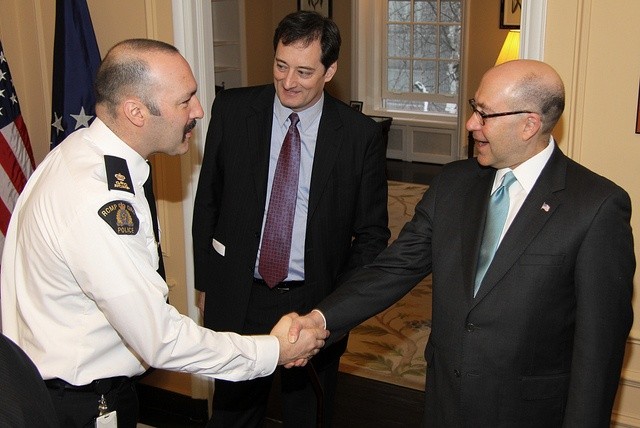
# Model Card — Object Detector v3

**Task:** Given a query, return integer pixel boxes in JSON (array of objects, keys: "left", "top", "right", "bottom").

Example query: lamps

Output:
[{"left": 495, "top": 29, "right": 522, "bottom": 64}]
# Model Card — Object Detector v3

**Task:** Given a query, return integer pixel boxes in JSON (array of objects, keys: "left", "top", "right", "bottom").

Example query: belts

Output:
[
  {"left": 255, "top": 278, "right": 305, "bottom": 294},
  {"left": 44, "top": 378, "right": 122, "bottom": 393}
]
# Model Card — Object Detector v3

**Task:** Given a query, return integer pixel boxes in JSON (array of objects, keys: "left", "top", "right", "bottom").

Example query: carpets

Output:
[{"left": 334, "top": 180, "right": 433, "bottom": 391}]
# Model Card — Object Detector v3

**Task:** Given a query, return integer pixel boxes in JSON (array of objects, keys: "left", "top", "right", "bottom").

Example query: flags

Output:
[
  {"left": 50, "top": 0, "right": 170, "bottom": 304},
  {"left": 0, "top": 41, "right": 37, "bottom": 261}
]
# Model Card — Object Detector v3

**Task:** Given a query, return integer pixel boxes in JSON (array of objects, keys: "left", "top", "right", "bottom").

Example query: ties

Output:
[
  {"left": 142, "top": 159, "right": 170, "bottom": 307},
  {"left": 257, "top": 112, "right": 302, "bottom": 291},
  {"left": 473, "top": 170, "right": 517, "bottom": 299}
]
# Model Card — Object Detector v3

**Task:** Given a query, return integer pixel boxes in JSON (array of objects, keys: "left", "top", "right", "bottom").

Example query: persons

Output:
[
  {"left": 1, "top": 40, "right": 332, "bottom": 428},
  {"left": 285, "top": 60, "right": 636, "bottom": 428},
  {"left": 191, "top": 10, "right": 391, "bottom": 428}
]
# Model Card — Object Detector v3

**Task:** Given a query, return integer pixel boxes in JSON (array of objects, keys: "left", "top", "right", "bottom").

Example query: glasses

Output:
[{"left": 469, "top": 98, "right": 542, "bottom": 125}]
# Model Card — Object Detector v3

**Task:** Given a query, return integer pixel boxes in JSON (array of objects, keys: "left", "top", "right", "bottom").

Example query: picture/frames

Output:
[
  {"left": 499, "top": 0, "right": 521, "bottom": 30},
  {"left": 297, "top": 0, "right": 334, "bottom": 21}
]
